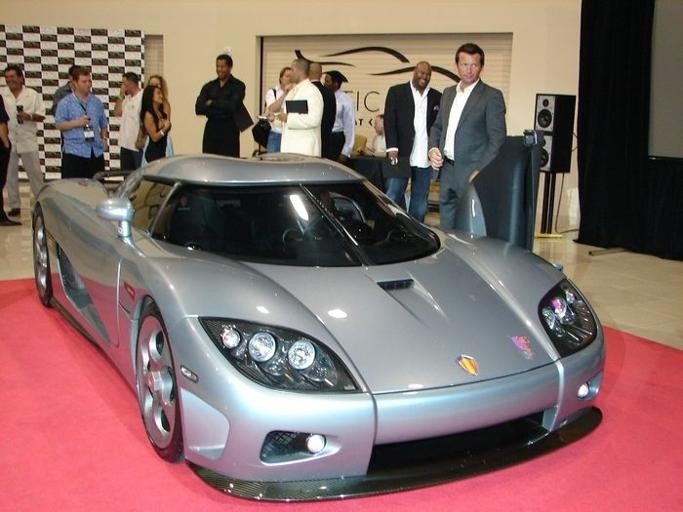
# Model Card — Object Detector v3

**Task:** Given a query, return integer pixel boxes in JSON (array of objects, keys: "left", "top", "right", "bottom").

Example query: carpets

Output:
[{"left": 1, "top": 275, "right": 683, "bottom": 507}]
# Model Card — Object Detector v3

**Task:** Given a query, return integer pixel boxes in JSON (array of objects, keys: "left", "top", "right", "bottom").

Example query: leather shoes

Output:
[
  {"left": 0, "top": 219, "right": 22, "bottom": 226},
  {"left": 8, "top": 208, "right": 20, "bottom": 217}
]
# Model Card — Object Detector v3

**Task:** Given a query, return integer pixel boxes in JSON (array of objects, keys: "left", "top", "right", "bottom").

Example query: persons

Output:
[
  {"left": 428, "top": 43, "right": 507, "bottom": 230},
  {"left": 268, "top": 59, "right": 324, "bottom": 158},
  {"left": 309, "top": 63, "right": 336, "bottom": 157},
  {"left": 114, "top": 72, "right": 144, "bottom": 170},
  {"left": 266, "top": 67, "right": 294, "bottom": 153},
  {"left": 0, "top": 94, "right": 22, "bottom": 227},
  {"left": 195, "top": 54, "right": 246, "bottom": 158},
  {"left": 324, "top": 70, "right": 355, "bottom": 163},
  {"left": 383, "top": 61, "right": 442, "bottom": 224},
  {"left": 141, "top": 75, "right": 174, "bottom": 167},
  {"left": 140, "top": 85, "right": 172, "bottom": 163},
  {"left": 0, "top": 66, "right": 45, "bottom": 216},
  {"left": 358, "top": 114, "right": 387, "bottom": 157},
  {"left": 53, "top": 65, "right": 82, "bottom": 114},
  {"left": 55, "top": 70, "right": 109, "bottom": 178}
]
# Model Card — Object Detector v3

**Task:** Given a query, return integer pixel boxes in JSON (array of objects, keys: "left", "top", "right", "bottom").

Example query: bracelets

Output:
[
  {"left": 160, "top": 130, "right": 164, "bottom": 136},
  {"left": 118, "top": 95, "right": 123, "bottom": 100}
]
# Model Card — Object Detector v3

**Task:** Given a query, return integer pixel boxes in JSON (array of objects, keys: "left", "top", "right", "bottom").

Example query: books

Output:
[
  {"left": 285, "top": 99, "right": 309, "bottom": 114},
  {"left": 233, "top": 103, "right": 253, "bottom": 132}
]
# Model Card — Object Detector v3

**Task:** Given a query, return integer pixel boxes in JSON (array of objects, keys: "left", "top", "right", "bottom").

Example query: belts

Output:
[{"left": 444, "top": 155, "right": 455, "bottom": 166}]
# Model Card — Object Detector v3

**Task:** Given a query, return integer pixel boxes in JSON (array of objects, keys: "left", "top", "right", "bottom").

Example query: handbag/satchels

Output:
[{"left": 252, "top": 88, "right": 276, "bottom": 149}]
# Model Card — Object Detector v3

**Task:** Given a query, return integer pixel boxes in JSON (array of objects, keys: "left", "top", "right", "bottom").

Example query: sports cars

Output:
[{"left": 29, "top": 150, "right": 607, "bottom": 504}]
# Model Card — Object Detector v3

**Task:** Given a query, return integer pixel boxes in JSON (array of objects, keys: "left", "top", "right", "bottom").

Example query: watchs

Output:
[{"left": 28, "top": 112, "right": 34, "bottom": 121}]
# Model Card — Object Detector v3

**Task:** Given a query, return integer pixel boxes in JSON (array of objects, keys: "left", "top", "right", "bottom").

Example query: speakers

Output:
[{"left": 533, "top": 93, "right": 576, "bottom": 174}]
